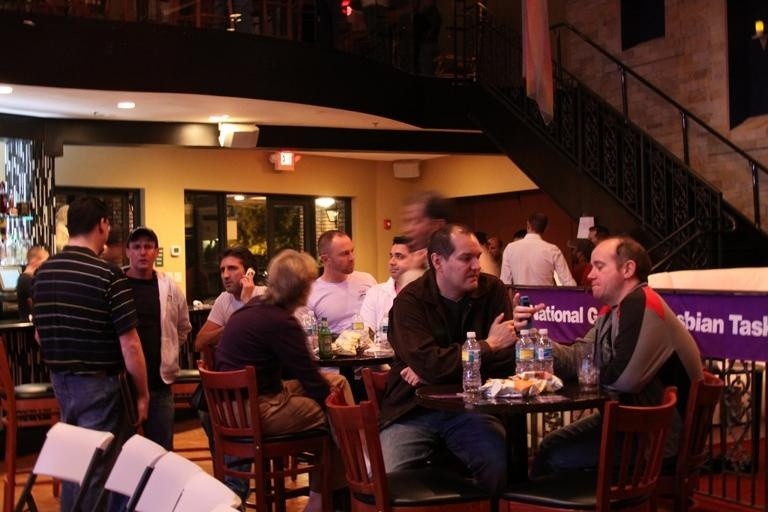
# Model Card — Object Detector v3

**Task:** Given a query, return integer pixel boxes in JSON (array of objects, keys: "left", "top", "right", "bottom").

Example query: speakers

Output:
[{"left": 218, "top": 124, "right": 260, "bottom": 148}]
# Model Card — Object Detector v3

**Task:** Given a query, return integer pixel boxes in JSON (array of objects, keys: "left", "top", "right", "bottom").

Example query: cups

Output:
[{"left": 573, "top": 343, "right": 599, "bottom": 392}]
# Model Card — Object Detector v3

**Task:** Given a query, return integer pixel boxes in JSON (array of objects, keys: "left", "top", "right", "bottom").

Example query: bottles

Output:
[
  {"left": 0, "top": 181, "right": 9, "bottom": 214},
  {"left": 532, "top": 329, "right": 555, "bottom": 373},
  {"left": 461, "top": 331, "right": 480, "bottom": 391},
  {"left": 303, "top": 309, "right": 388, "bottom": 360},
  {"left": 515, "top": 328, "right": 532, "bottom": 372}
]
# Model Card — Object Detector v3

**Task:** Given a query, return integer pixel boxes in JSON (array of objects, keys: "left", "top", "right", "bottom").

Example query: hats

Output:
[{"left": 127, "top": 227, "right": 158, "bottom": 247}]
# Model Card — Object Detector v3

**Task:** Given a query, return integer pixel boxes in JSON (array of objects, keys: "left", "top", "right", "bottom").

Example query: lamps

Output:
[{"left": 270, "top": 151, "right": 296, "bottom": 172}]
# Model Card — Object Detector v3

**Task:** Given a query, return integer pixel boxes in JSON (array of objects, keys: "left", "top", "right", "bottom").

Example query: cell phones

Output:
[
  {"left": 245, "top": 268, "right": 256, "bottom": 278},
  {"left": 520, "top": 296, "right": 532, "bottom": 330}
]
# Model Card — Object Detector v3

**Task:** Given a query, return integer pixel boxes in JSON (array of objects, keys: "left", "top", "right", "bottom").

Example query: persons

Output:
[
  {"left": 192, "top": 246, "right": 270, "bottom": 500},
  {"left": 29, "top": 195, "right": 153, "bottom": 511},
  {"left": 299, "top": 189, "right": 623, "bottom": 388},
  {"left": 207, "top": 247, "right": 359, "bottom": 512},
  {"left": 15, "top": 245, "right": 51, "bottom": 322},
  {"left": 118, "top": 224, "right": 193, "bottom": 455},
  {"left": 511, "top": 232, "right": 708, "bottom": 499},
  {"left": 359, "top": 224, "right": 532, "bottom": 503}
]
